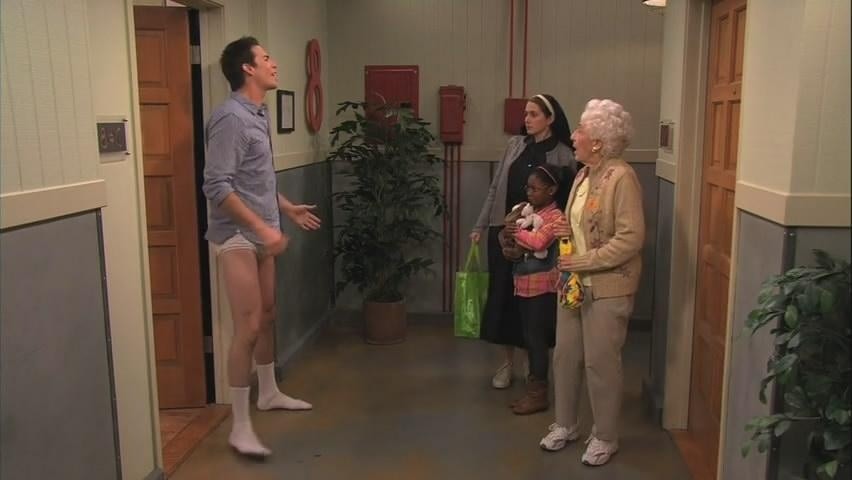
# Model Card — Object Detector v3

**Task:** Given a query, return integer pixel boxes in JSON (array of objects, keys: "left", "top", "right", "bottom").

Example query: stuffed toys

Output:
[
  {"left": 497, "top": 201, "right": 531, "bottom": 262},
  {"left": 514, "top": 203, "right": 549, "bottom": 260}
]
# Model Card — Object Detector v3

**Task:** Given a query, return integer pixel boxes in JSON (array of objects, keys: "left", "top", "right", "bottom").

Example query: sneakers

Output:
[
  {"left": 582, "top": 433, "right": 618, "bottom": 465},
  {"left": 540, "top": 416, "right": 580, "bottom": 451},
  {"left": 493, "top": 361, "right": 513, "bottom": 389}
]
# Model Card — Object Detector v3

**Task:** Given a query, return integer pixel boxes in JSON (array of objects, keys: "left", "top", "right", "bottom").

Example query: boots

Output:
[{"left": 508, "top": 373, "right": 548, "bottom": 414}]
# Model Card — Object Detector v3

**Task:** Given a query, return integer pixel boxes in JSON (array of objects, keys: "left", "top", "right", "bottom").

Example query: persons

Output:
[
  {"left": 537, "top": 98, "right": 647, "bottom": 468},
  {"left": 201, "top": 36, "right": 322, "bottom": 458},
  {"left": 498, "top": 161, "right": 567, "bottom": 416},
  {"left": 472, "top": 91, "right": 581, "bottom": 388}
]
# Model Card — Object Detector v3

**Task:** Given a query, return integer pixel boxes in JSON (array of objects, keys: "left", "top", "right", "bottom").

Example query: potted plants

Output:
[{"left": 325, "top": 89, "right": 451, "bottom": 347}]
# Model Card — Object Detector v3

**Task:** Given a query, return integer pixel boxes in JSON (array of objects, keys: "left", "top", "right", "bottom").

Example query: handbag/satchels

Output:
[
  {"left": 455, "top": 270, "right": 490, "bottom": 338},
  {"left": 559, "top": 274, "right": 585, "bottom": 310}
]
